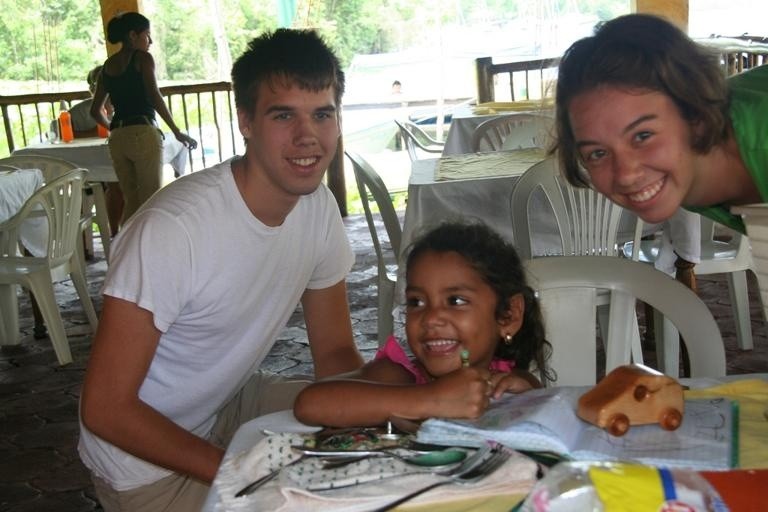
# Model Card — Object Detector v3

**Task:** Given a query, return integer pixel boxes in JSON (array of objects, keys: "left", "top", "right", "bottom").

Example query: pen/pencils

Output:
[{"left": 461, "top": 350, "right": 470, "bottom": 367}]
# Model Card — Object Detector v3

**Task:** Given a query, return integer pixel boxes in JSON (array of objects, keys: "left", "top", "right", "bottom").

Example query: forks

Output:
[
  {"left": 371, "top": 443, "right": 514, "bottom": 512},
  {"left": 306, "top": 443, "right": 492, "bottom": 498}
]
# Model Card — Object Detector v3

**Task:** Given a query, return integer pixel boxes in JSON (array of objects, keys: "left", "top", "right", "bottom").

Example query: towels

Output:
[{"left": 234, "top": 422, "right": 537, "bottom": 512}]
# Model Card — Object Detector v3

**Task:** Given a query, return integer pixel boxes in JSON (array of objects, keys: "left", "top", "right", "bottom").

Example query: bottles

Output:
[
  {"left": 59, "top": 99, "right": 73, "bottom": 142},
  {"left": 97, "top": 103, "right": 108, "bottom": 137}
]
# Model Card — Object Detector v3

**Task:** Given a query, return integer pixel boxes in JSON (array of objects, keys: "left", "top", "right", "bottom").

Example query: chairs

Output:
[
  {"left": 627, "top": 218, "right": 760, "bottom": 373},
  {"left": 522, "top": 255, "right": 726, "bottom": 381},
  {"left": 510, "top": 158, "right": 643, "bottom": 375},
  {"left": 344, "top": 150, "right": 404, "bottom": 350},
  {"left": 394, "top": 117, "right": 445, "bottom": 160},
  {"left": 715, "top": 44, "right": 768, "bottom": 78},
  {"left": 1, "top": 166, "right": 98, "bottom": 366},
  {"left": 478, "top": 56, "right": 561, "bottom": 100},
  {"left": 472, "top": 113, "right": 558, "bottom": 153},
  {"left": 1, "top": 156, "right": 112, "bottom": 267}
]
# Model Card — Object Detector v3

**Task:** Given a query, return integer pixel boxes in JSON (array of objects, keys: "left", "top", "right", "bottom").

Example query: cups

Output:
[{"left": 48, "top": 119, "right": 59, "bottom": 143}]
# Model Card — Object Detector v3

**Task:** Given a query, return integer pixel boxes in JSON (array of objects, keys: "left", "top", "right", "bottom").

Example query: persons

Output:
[
  {"left": 76, "top": 28, "right": 364, "bottom": 512},
  {"left": 294, "top": 218, "right": 558, "bottom": 428},
  {"left": 90, "top": 11, "right": 197, "bottom": 232},
  {"left": 54, "top": 66, "right": 125, "bottom": 264},
  {"left": 554, "top": 14, "right": 768, "bottom": 241},
  {"left": 391, "top": 80, "right": 403, "bottom": 97}
]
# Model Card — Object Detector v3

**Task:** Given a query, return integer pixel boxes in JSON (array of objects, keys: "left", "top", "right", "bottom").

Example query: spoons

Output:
[{"left": 290, "top": 444, "right": 467, "bottom": 467}]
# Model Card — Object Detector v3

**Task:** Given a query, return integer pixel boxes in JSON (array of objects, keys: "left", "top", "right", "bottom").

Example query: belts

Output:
[{"left": 109, "top": 117, "right": 156, "bottom": 129}]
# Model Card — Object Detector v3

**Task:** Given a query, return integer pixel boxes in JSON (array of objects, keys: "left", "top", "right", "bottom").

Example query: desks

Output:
[
  {"left": 441, "top": 98, "right": 561, "bottom": 157},
  {"left": 11, "top": 131, "right": 190, "bottom": 261},
  {"left": 1, "top": 165, "right": 45, "bottom": 259},
  {"left": 401, "top": 148, "right": 702, "bottom": 294},
  {"left": 201, "top": 374, "right": 768, "bottom": 512}
]
